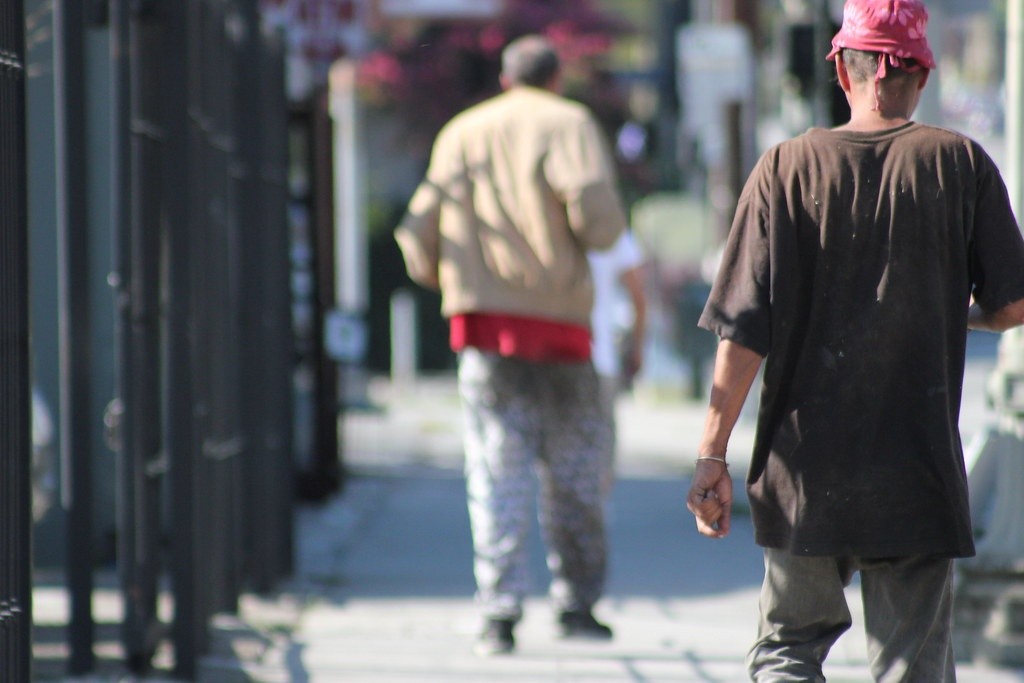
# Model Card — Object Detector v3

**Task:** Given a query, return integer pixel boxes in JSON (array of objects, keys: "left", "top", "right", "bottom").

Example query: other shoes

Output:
[
  {"left": 472, "top": 620, "right": 514, "bottom": 656},
  {"left": 554, "top": 611, "right": 613, "bottom": 641}
]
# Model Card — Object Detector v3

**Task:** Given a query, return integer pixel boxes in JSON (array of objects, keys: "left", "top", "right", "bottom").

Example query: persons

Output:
[
  {"left": 683, "top": 0, "right": 1024, "bottom": 683},
  {"left": 394, "top": 35, "right": 645, "bottom": 657}
]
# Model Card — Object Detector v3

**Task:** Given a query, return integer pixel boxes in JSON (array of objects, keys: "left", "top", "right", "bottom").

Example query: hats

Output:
[{"left": 827, "top": 0, "right": 935, "bottom": 112}]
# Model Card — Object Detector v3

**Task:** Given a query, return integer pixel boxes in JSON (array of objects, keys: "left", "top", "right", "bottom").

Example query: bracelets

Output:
[{"left": 695, "top": 457, "right": 726, "bottom": 464}]
